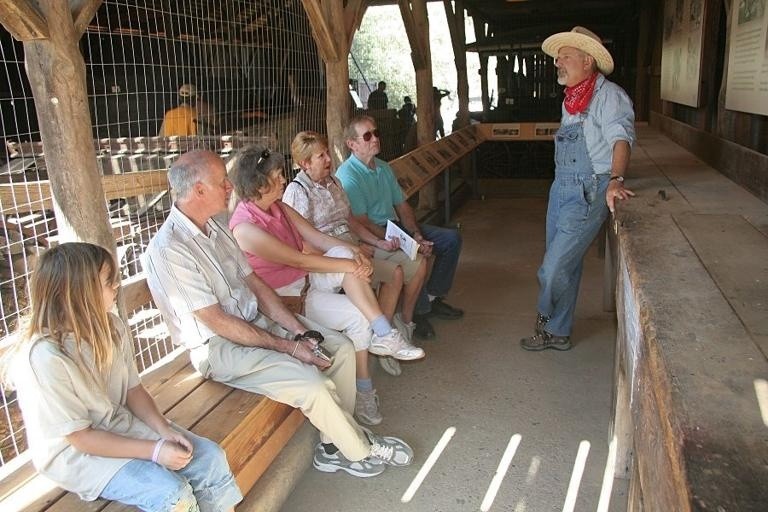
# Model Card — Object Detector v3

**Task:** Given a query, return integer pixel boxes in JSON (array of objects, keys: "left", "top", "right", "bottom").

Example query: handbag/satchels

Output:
[{"left": 278, "top": 295, "right": 305, "bottom": 319}]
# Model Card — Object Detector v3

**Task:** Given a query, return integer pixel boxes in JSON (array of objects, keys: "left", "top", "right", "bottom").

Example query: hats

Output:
[
  {"left": 179, "top": 84, "right": 199, "bottom": 99},
  {"left": 542, "top": 27, "right": 614, "bottom": 76}
]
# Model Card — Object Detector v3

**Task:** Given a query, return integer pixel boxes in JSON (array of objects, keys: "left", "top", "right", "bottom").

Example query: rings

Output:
[{"left": 364, "top": 266, "right": 369, "bottom": 270}]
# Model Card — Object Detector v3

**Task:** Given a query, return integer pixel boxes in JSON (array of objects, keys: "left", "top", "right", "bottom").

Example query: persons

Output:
[
  {"left": 3, "top": 242, "right": 245, "bottom": 511},
  {"left": 403, "top": 96, "right": 415, "bottom": 115},
  {"left": 368, "top": 81, "right": 389, "bottom": 110},
  {"left": 452, "top": 111, "right": 461, "bottom": 132},
  {"left": 518, "top": 25, "right": 636, "bottom": 351},
  {"left": 158, "top": 83, "right": 210, "bottom": 137},
  {"left": 433, "top": 87, "right": 450, "bottom": 139},
  {"left": 281, "top": 131, "right": 432, "bottom": 377},
  {"left": 333, "top": 113, "right": 464, "bottom": 339},
  {"left": 228, "top": 143, "right": 426, "bottom": 428},
  {"left": 141, "top": 149, "right": 415, "bottom": 478}
]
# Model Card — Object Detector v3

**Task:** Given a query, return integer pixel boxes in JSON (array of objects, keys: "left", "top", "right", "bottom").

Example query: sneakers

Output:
[
  {"left": 368, "top": 330, "right": 426, "bottom": 361},
  {"left": 429, "top": 298, "right": 464, "bottom": 319},
  {"left": 536, "top": 311, "right": 550, "bottom": 334},
  {"left": 414, "top": 316, "right": 435, "bottom": 342},
  {"left": 354, "top": 387, "right": 383, "bottom": 424},
  {"left": 391, "top": 313, "right": 416, "bottom": 342},
  {"left": 358, "top": 425, "right": 415, "bottom": 468},
  {"left": 520, "top": 331, "right": 572, "bottom": 352},
  {"left": 312, "top": 442, "right": 385, "bottom": 478},
  {"left": 378, "top": 356, "right": 402, "bottom": 377}
]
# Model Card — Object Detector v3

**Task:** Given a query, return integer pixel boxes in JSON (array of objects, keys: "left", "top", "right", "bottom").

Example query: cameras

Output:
[{"left": 312, "top": 345, "right": 332, "bottom": 361}]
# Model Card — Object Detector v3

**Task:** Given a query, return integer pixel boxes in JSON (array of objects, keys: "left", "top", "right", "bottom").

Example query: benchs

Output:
[{"left": 0, "top": 273, "right": 306, "bottom": 512}]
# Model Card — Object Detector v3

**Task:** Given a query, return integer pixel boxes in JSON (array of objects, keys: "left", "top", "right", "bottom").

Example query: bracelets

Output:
[
  {"left": 291, "top": 340, "right": 300, "bottom": 358},
  {"left": 152, "top": 439, "right": 165, "bottom": 463},
  {"left": 410, "top": 229, "right": 420, "bottom": 237}
]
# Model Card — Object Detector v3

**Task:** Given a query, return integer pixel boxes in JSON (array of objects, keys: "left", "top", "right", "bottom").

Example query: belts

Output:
[{"left": 325, "top": 223, "right": 351, "bottom": 237}]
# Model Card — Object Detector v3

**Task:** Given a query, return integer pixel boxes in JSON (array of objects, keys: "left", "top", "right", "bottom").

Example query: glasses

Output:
[
  {"left": 354, "top": 128, "right": 379, "bottom": 142},
  {"left": 256, "top": 148, "right": 272, "bottom": 165},
  {"left": 294, "top": 330, "right": 324, "bottom": 344}
]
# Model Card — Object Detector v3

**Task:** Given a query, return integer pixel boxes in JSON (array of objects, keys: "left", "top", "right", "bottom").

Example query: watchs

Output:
[{"left": 609, "top": 175, "right": 624, "bottom": 183}]
